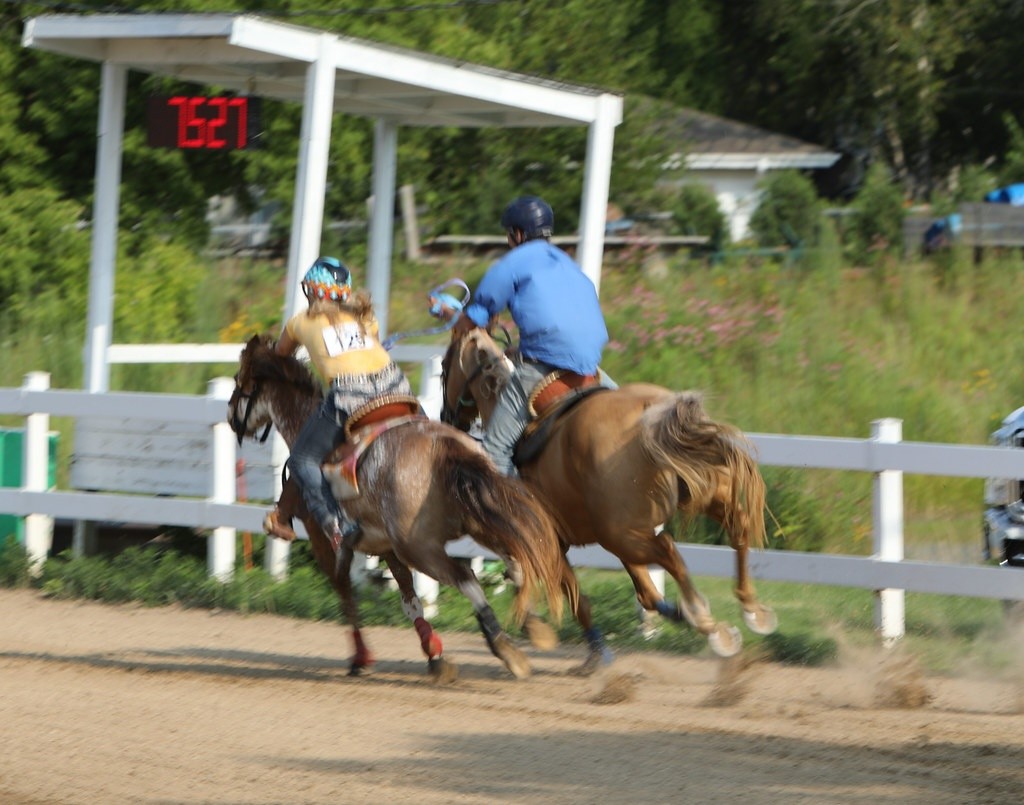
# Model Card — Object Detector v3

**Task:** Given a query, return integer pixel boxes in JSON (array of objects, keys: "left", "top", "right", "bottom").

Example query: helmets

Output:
[
  {"left": 304, "top": 256, "right": 352, "bottom": 304},
  {"left": 501, "top": 195, "right": 554, "bottom": 240}
]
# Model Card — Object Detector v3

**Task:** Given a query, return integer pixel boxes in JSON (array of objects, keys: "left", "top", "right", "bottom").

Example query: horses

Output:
[
  {"left": 438, "top": 301, "right": 782, "bottom": 658},
  {"left": 227, "top": 313, "right": 609, "bottom": 691}
]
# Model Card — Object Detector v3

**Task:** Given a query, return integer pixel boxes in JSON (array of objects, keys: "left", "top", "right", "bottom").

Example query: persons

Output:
[
  {"left": 275, "top": 257, "right": 413, "bottom": 572},
  {"left": 429, "top": 195, "right": 609, "bottom": 476}
]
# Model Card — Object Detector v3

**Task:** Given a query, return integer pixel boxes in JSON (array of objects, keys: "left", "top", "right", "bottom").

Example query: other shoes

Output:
[{"left": 321, "top": 508, "right": 365, "bottom": 581}]
